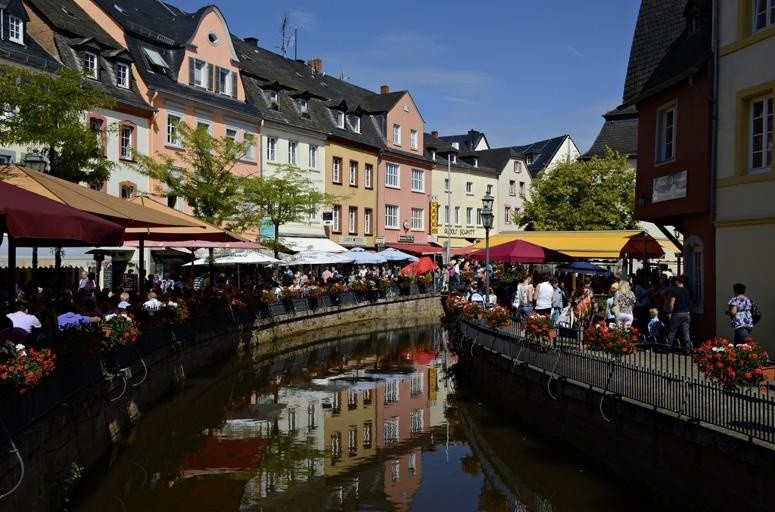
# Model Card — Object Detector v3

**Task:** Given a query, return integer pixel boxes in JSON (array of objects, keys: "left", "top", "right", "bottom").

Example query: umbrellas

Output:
[
  {"left": 0, "top": 157, "right": 211, "bottom": 235},
  {"left": 134, "top": 213, "right": 421, "bottom": 270},
  {"left": 115, "top": 192, "right": 252, "bottom": 296},
  {"left": 462, "top": 239, "right": 581, "bottom": 264},
  {"left": 0, "top": 179, "right": 127, "bottom": 249}
]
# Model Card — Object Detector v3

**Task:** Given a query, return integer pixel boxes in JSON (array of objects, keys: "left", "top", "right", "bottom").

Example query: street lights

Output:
[{"left": 480, "top": 190, "right": 494, "bottom": 307}]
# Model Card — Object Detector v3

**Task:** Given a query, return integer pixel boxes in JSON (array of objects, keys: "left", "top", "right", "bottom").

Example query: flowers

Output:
[
  {"left": 1, "top": 277, "right": 437, "bottom": 387},
  {"left": 688, "top": 336, "right": 768, "bottom": 388},
  {"left": 440, "top": 277, "right": 643, "bottom": 359}
]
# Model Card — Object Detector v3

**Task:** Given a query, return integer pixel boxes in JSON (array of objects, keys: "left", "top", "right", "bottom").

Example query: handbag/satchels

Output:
[
  {"left": 511, "top": 288, "right": 521, "bottom": 309},
  {"left": 751, "top": 305, "right": 764, "bottom": 326}
]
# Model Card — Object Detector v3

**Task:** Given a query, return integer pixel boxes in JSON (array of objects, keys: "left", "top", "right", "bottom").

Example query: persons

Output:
[
  {"left": 1, "top": 258, "right": 698, "bottom": 380},
  {"left": 725, "top": 281, "right": 757, "bottom": 347},
  {"left": 515, "top": 266, "right": 697, "bottom": 355},
  {"left": 405, "top": 259, "right": 500, "bottom": 311},
  {"left": 238, "top": 262, "right": 403, "bottom": 295},
  {"left": 1, "top": 267, "right": 237, "bottom": 346}
]
